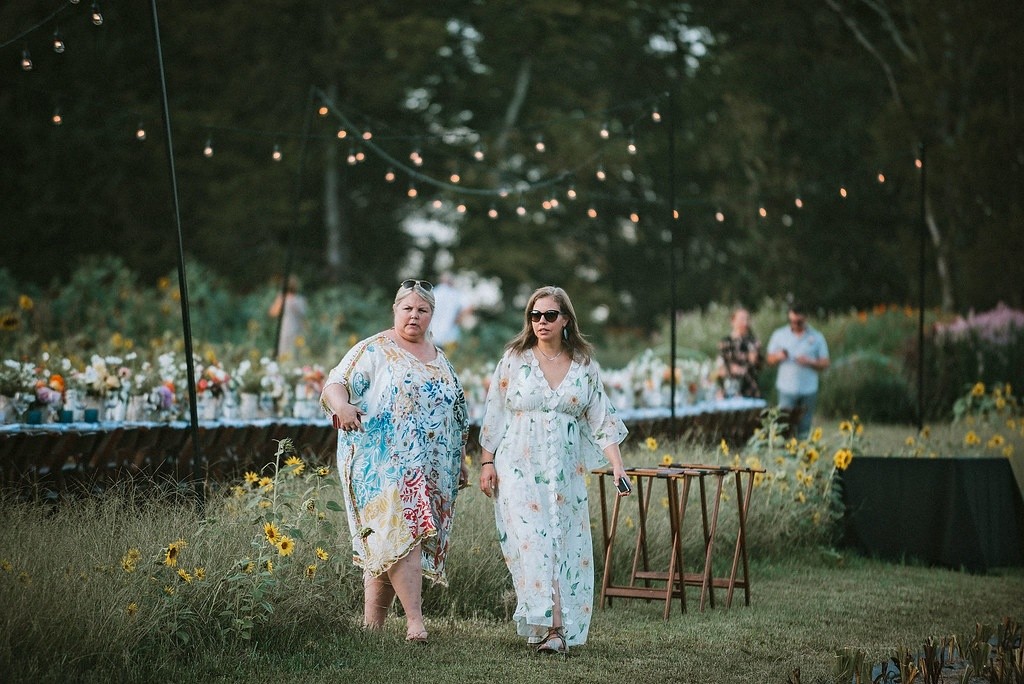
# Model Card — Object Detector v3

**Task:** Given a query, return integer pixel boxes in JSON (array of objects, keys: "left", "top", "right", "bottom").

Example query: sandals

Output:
[{"left": 537, "top": 626, "right": 569, "bottom": 654}]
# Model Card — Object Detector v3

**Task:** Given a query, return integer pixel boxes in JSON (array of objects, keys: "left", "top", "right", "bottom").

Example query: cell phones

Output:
[{"left": 613, "top": 477, "right": 631, "bottom": 494}]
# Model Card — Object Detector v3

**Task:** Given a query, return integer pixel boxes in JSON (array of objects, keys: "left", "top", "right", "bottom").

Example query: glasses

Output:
[
  {"left": 528, "top": 310, "right": 566, "bottom": 323},
  {"left": 401, "top": 279, "right": 434, "bottom": 293}
]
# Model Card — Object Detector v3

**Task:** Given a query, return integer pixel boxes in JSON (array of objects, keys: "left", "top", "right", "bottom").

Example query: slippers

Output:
[{"left": 406, "top": 630, "right": 427, "bottom": 641}]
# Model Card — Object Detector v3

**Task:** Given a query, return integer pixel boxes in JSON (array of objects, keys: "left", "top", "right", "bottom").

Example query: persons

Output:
[
  {"left": 428, "top": 268, "right": 472, "bottom": 361},
  {"left": 478, "top": 284, "right": 634, "bottom": 653},
  {"left": 764, "top": 302, "right": 832, "bottom": 443},
  {"left": 318, "top": 278, "right": 471, "bottom": 644},
  {"left": 269, "top": 272, "right": 309, "bottom": 362},
  {"left": 717, "top": 307, "right": 764, "bottom": 446}
]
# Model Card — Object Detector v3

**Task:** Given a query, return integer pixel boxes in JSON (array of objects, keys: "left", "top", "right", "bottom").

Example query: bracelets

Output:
[{"left": 482, "top": 461, "right": 495, "bottom": 467}]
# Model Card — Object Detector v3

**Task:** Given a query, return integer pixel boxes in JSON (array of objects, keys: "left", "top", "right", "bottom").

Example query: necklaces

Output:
[{"left": 535, "top": 344, "right": 566, "bottom": 361}]
